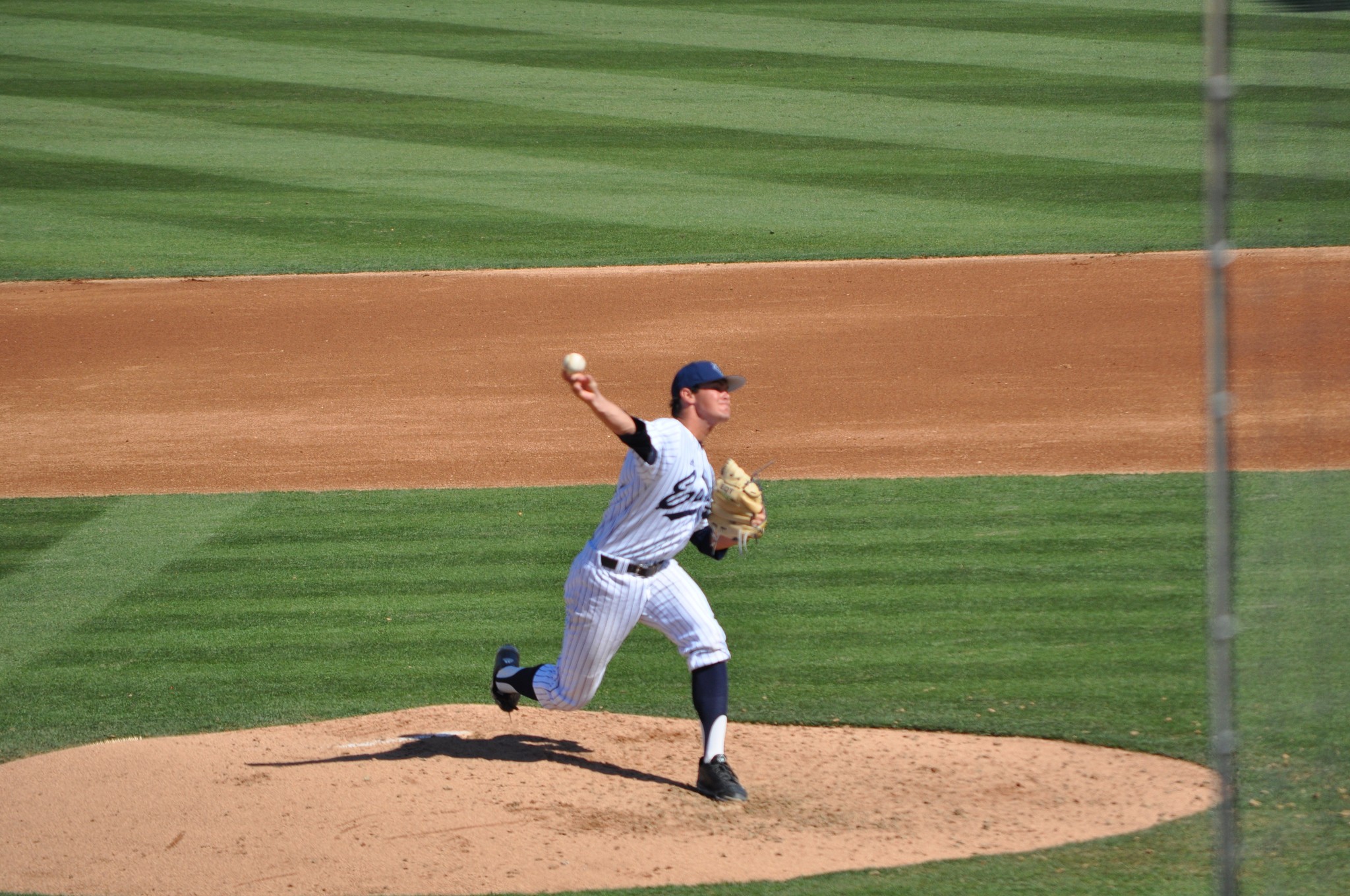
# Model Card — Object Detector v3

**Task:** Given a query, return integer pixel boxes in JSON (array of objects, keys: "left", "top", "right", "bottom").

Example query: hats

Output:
[{"left": 671, "top": 361, "right": 747, "bottom": 397}]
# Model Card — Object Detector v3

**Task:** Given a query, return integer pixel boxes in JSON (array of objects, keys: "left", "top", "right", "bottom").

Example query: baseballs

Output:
[{"left": 563, "top": 353, "right": 586, "bottom": 372}]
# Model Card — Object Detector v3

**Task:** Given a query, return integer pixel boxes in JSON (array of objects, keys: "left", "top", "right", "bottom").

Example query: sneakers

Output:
[
  {"left": 490, "top": 645, "right": 520, "bottom": 713},
  {"left": 696, "top": 754, "right": 747, "bottom": 802}
]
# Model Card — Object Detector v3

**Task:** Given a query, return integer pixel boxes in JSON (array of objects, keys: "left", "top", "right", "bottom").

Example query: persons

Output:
[{"left": 492, "top": 359, "right": 767, "bottom": 804}]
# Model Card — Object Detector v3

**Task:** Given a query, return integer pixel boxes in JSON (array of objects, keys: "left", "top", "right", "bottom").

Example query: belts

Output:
[{"left": 600, "top": 553, "right": 666, "bottom": 577}]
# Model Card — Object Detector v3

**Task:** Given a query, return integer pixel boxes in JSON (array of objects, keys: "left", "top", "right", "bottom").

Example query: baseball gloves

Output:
[{"left": 708, "top": 457, "right": 766, "bottom": 542}]
